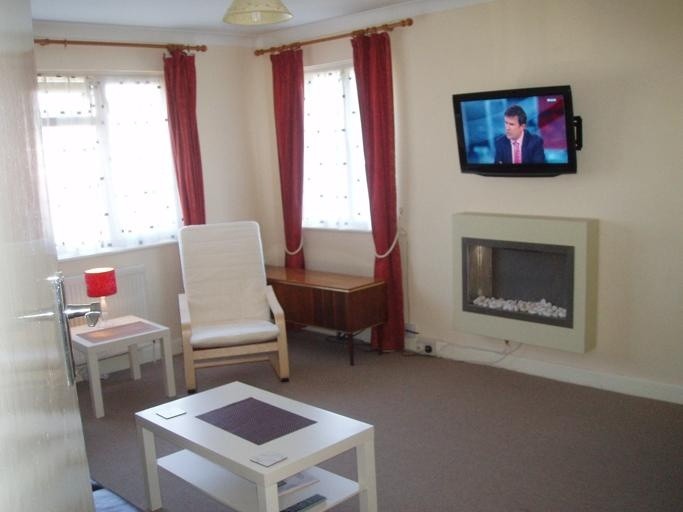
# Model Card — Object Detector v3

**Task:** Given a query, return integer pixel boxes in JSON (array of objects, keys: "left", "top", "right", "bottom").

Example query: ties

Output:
[{"left": 512, "top": 141, "right": 521, "bottom": 164}]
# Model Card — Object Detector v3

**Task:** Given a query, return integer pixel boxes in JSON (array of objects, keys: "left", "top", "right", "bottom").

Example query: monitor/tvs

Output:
[{"left": 452, "top": 85, "right": 582, "bottom": 178}]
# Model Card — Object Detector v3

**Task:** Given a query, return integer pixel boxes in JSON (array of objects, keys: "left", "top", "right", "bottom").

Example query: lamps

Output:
[
  {"left": 222, "top": 1, "right": 293, "bottom": 26},
  {"left": 86, "top": 268, "right": 120, "bottom": 322}
]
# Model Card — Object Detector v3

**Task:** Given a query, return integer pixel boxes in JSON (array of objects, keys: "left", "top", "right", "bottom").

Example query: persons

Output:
[{"left": 495, "top": 105, "right": 545, "bottom": 164}]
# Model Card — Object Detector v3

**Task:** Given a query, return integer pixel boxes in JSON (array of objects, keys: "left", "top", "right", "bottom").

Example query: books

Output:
[{"left": 278, "top": 470, "right": 320, "bottom": 496}]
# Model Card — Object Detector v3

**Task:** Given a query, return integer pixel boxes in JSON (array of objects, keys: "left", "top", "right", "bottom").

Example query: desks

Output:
[
  {"left": 266, "top": 265, "right": 388, "bottom": 365},
  {"left": 72, "top": 314, "right": 178, "bottom": 419},
  {"left": 136, "top": 379, "right": 377, "bottom": 511}
]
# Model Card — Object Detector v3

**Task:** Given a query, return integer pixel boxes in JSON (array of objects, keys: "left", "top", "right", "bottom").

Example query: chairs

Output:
[{"left": 176, "top": 219, "right": 291, "bottom": 394}]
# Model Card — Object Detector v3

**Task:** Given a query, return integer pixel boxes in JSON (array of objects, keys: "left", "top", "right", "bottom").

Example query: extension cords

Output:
[{"left": 327, "top": 336, "right": 363, "bottom": 345}]
